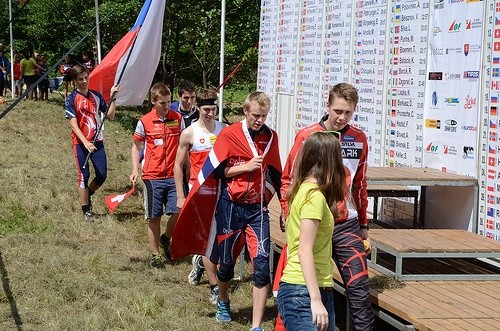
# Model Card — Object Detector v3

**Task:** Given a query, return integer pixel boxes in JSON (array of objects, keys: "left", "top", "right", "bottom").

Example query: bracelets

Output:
[{"left": 360, "top": 225, "right": 369, "bottom": 230}]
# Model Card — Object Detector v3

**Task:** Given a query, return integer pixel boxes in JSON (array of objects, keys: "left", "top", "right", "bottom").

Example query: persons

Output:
[
  {"left": 130, "top": 83, "right": 192, "bottom": 269},
  {"left": 212, "top": 91, "right": 278, "bottom": 331},
  {"left": 0, "top": 43, "right": 9, "bottom": 96},
  {"left": 280, "top": 83, "right": 378, "bottom": 331},
  {"left": 65, "top": 65, "right": 120, "bottom": 218},
  {"left": 170, "top": 82, "right": 200, "bottom": 127},
  {"left": 14, "top": 51, "right": 50, "bottom": 101},
  {"left": 173, "top": 88, "right": 230, "bottom": 306},
  {"left": 83, "top": 51, "right": 94, "bottom": 73},
  {"left": 277, "top": 132, "right": 347, "bottom": 331},
  {"left": 60, "top": 56, "right": 74, "bottom": 98}
]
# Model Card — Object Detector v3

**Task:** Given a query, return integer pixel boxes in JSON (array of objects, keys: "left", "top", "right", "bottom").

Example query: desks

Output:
[{"left": 268, "top": 165, "right": 500, "bottom": 331}]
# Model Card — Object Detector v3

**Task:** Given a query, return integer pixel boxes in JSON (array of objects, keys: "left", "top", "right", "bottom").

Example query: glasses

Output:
[{"left": 311, "top": 131, "right": 341, "bottom": 143}]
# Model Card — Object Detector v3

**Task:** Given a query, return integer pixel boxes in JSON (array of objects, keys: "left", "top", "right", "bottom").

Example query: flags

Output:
[
  {"left": 105, "top": 187, "right": 134, "bottom": 213},
  {"left": 89, "top": 0, "right": 164, "bottom": 106}
]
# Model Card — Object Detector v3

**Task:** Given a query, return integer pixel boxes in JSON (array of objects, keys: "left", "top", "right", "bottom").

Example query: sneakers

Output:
[
  {"left": 151, "top": 254, "right": 162, "bottom": 269},
  {"left": 211, "top": 286, "right": 219, "bottom": 304},
  {"left": 83, "top": 211, "right": 93, "bottom": 219},
  {"left": 188, "top": 255, "right": 205, "bottom": 286},
  {"left": 216, "top": 300, "right": 232, "bottom": 322},
  {"left": 159, "top": 233, "right": 174, "bottom": 263},
  {"left": 249, "top": 327, "right": 264, "bottom": 331}
]
